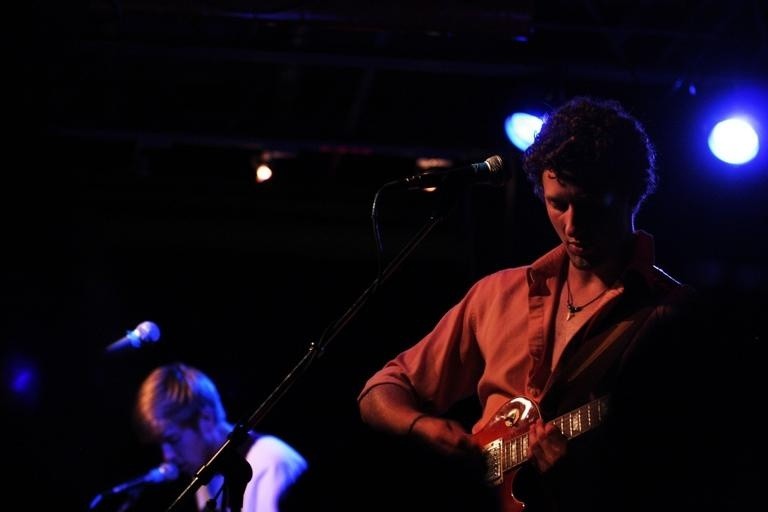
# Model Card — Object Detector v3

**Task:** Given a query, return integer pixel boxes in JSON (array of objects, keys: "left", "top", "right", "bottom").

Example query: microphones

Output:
[
  {"left": 405, "top": 155, "right": 502, "bottom": 189},
  {"left": 106, "top": 319, "right": 160, "bottom": 349}
]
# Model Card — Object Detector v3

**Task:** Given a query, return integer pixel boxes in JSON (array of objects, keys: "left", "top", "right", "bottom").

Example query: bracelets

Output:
[{"left": 407, "top": 414, "right": 425, "bottom": 432}]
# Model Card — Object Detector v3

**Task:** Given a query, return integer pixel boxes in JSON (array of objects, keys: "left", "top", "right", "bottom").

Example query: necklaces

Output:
[{"left": 563, "top": 276, "right": 608, "bottom": 319}]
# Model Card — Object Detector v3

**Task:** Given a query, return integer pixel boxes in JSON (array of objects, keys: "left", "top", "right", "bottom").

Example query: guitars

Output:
[{"left": 465, "top": 396, "right": 609, "bottom": 512}]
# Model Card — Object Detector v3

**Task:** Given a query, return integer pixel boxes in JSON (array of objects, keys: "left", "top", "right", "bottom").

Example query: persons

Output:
[
  {"left": 353, "top": 90, "right": 707, "bottom": 512},
  {"left": 133, "top": 361, "right": 308, "bottom": 512}
]
